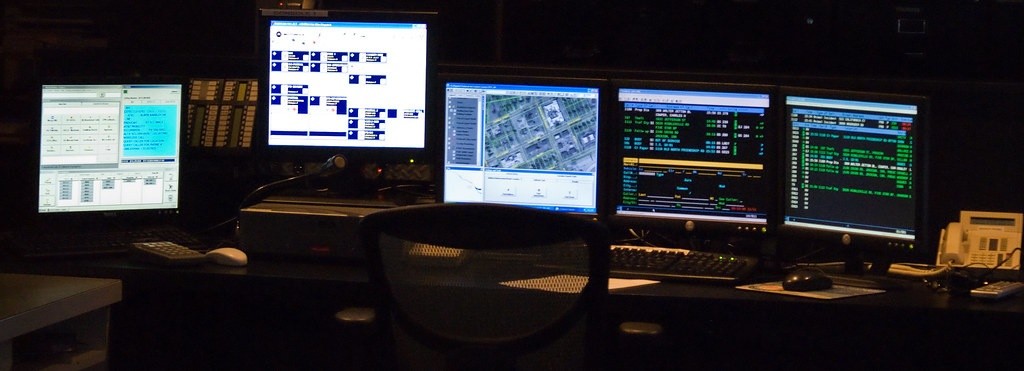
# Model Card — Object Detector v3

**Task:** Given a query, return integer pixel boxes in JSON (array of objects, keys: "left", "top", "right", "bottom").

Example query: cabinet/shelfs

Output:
[{"left": 0, "top": 272, "right": 123, "bottom": 371}]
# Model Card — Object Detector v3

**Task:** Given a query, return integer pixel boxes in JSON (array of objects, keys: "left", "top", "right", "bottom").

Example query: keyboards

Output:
[
  {"left": 3, "top": 224, "right": 210, "bottom": 258},
  {"left": 533, "top": 241, "right": 767, "bottom": 284}
]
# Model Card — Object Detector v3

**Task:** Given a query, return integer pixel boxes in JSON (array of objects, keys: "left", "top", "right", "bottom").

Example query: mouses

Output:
[
  {"left": 783, "top": 269, "right": 834, "bottom": 292},
  {"left": 205, "top": 247, "right": 247, "bottom": 266}
]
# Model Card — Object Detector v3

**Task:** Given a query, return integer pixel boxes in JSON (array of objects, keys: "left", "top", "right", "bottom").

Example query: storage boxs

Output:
[{"left": 237, "top": 195, "right": 398, "bottom": 259}]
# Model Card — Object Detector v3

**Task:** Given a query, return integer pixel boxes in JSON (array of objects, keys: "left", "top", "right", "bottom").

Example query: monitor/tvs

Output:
[
  {"left": 436, "top": 62, "right": 929, "bottom": 291},
  {"left": 257, "top": 8, "right": 439, "bottom": 197},
  {"left": 32, "top": 76, "right": 190, "bottom": 217}
]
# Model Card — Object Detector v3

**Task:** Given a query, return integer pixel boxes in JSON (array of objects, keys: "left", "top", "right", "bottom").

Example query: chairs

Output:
[{"left": 358, "top": 202, "right": 611, "bottom": 371}]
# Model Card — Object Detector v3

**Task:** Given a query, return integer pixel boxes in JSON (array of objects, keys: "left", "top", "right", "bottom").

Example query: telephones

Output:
[{"left": 936, "top": 211, "right": 1023, "bottom": 282}]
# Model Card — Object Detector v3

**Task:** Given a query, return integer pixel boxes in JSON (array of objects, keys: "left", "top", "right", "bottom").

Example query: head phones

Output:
[{"left": 940, "top": 269, "right": 988, "bottom": 297}]
